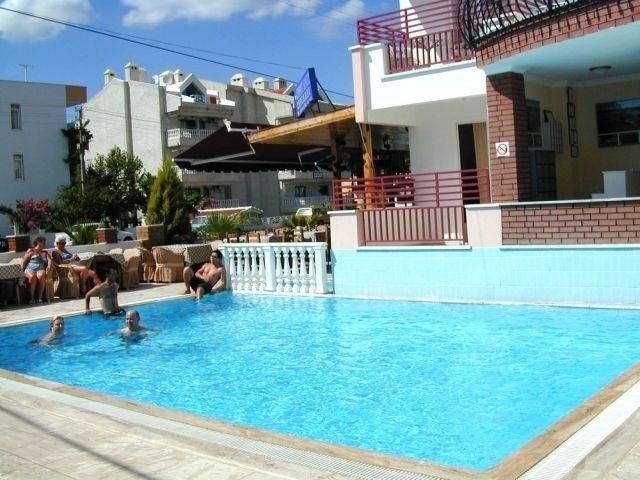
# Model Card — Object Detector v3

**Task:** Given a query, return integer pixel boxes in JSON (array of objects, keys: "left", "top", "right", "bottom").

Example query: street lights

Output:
[{"left": 74, "top": 107, "right": 91, "bottom": 196}]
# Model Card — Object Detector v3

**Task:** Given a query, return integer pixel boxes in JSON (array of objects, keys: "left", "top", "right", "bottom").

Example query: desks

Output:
[
  {"left": 160, "top": 243, "right": 212, "bottom": 272},
  {"left": 602, "top": 171, "right": 631, "bottom": 198}
]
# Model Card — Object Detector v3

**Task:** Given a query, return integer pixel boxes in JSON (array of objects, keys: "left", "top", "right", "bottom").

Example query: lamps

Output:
[{"left": 589, "top": 66, "right": 611, "bottom": 74}]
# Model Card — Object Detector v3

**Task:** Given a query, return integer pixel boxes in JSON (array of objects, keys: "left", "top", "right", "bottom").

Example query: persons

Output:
[
  {"left": 181, "top": 248, "right": 226, "bottom": 303},
  {"left": 107, "top": 307, "right": 157, "bottom": 350},
  {"left": 84, "top": 267, "right": 127, "bottom": 318},
  {"left": 52, "top": 235, "right": 102, "bottom": 296},
  {"left": 25, "top": 315, "right": 77, "bottom": 347},
  {"left": 21, "top": 236, "right": 53, "bottom": 304}
]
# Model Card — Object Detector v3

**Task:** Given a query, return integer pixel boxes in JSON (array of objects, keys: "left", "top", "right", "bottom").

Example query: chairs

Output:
[
  {"left": 0, "top": 247, "right": 185, "bottom": 305},
  {"left": 210, "top": 240, "right": 223, "bottom": 252}
]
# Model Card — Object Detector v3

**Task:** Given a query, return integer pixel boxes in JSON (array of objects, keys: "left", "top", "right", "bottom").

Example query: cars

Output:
[
  {"left": 54, "top": 223, "right": 136, "bottom": 247},
  {"left": 295, "top": 207, "right": 330, "bottom": 225},
  {"left": 190, "top": 215, "right": 245, "bottom": 238}
]
